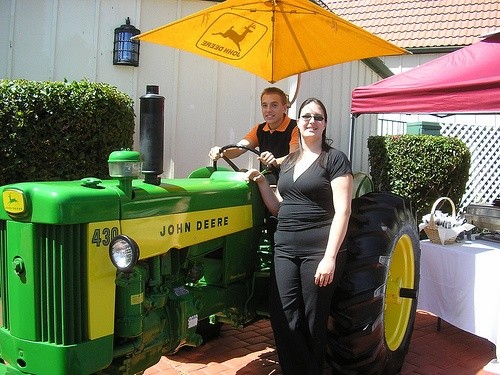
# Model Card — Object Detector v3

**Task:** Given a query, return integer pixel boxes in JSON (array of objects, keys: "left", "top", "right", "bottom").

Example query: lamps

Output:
[{"left": 113, "top": 16, "right": 141, "bottom": 67}]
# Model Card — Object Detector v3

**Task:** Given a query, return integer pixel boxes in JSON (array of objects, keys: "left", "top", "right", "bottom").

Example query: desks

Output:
[{"left": 420, "top": 240, "right": 500, "bottom": 362}]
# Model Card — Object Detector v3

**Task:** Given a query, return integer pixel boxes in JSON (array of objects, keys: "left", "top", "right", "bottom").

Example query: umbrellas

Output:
[{"left": 129, "top": 0, "right": 414, "bottom": 86}]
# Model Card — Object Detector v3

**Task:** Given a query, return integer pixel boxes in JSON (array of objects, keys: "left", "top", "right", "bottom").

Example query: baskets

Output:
[{"left": 423, "top": 197, "right": 459, "bottom": 245}]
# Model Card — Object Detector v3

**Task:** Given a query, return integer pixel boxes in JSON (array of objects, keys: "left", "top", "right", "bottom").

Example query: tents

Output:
[{"left": 349, "top": 33, "right": 500, "bottom": 173}]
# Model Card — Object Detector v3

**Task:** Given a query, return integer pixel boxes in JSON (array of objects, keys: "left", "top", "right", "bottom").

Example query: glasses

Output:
[{"left": 299, "top": 114, "right": 325, "bottom": 121}]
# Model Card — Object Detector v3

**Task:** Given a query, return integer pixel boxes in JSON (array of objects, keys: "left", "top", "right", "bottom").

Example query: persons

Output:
[
  {"left": 244, "top": 97, "right": 353, "bottom": 375},
  {"left": 208, "top": 87, "right": 299, "bottom": 221}
]
